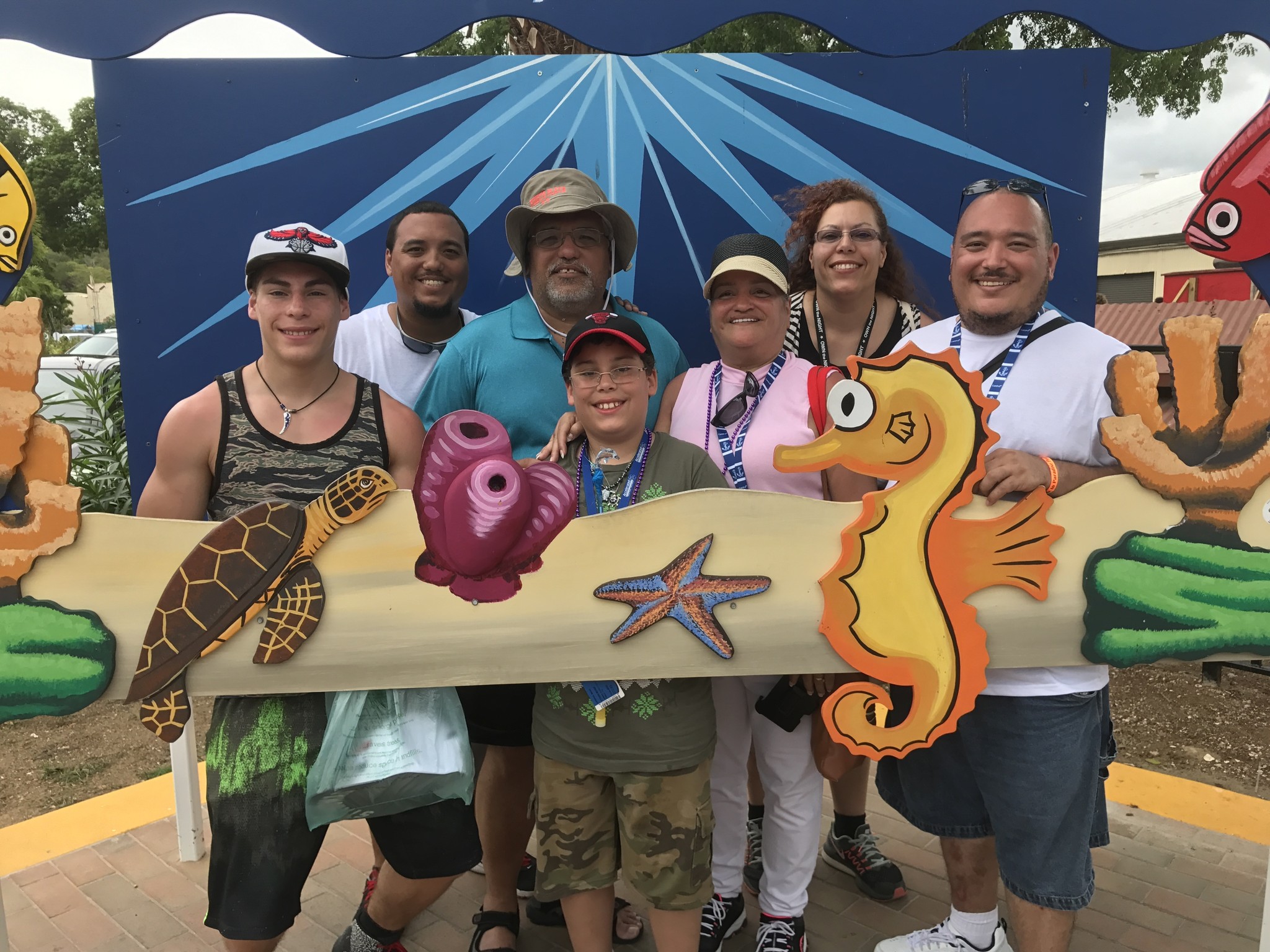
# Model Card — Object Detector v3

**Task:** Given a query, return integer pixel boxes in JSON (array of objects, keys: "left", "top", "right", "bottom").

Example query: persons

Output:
[
  {"left": 743, "top": 179, "right": 937, "bottom": 900},
  {"left": 138, "top": 221, "right": 485, "bottom": 952},
  {"left": 864, "top": 177, "right": 1139, "bottom": 952},
  {"left": 535, "top": 234, "right": 880, "bottom": 952},
  {"left": 333, "top": 204, "right": 647, "bottom": 926},
  {"left": 408, "top": 169, "right": 697, "bottom": 952}
]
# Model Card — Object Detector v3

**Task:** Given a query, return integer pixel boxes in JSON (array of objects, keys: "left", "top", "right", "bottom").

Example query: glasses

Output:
[
  {"left": 532, "top": 226, "right": 613, "bottom": 250},
  {"left": 711, "top": 369, "right": 759, "bottom": 428},
  {"left": 568, "top": 366, "right": 647, "bottom": 389},
  {"left": 812, "top": 228, "right": 881, "bottom": 242},
  {"left": 956, "top": 178, "right": 1052, "bottom": 236}
]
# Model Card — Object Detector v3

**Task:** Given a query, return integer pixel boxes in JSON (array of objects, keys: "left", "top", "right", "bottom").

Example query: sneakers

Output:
[
  {"left": 694, "top": 892, "right": 748, "bottom": 952},
  {"left": 743, "top": 815, "right": 767, "bottom": 895},
  {"left": 875, "top": 914, "right": 1017, "bottom": 952},
  {"left": 753, "top": 910, "right": 808, "bottom": 952},
  {"left": 822, "top": 814, "right": 910, "bottom": 903}
]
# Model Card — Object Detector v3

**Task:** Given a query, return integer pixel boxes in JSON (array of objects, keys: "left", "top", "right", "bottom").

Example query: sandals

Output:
[
  {"left": 526, "top": 885, "right": 644, "bottom": 944},
  {"left": 470, "top": 902, "right": 520, "bottom": 952}
]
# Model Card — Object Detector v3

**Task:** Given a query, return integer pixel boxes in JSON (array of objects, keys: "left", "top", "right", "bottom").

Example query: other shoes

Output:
[
  {"left": 330, "top": 921, "right": 408, "bottom": 952},
  {"left": 356, "top": 866, "right": 385, "bottom": 919},
  {"left": 472, "top": 845, "right": 538, "bottom": 886}
]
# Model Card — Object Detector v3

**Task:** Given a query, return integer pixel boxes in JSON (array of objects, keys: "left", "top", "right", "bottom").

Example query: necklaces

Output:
[
  {"left": 256, "top": 355, "right": 340, "bottom": 434},
  {"left": 705, "top": 361, "right": 758, "bottom": 476},
  {"left": 575, "top": 427, "right": 652, "bottom": 517}
]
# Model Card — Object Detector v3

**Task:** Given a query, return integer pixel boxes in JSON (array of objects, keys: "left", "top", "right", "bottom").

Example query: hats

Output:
[
  {"left": 561, "top": 313, "right": 655, "bottom": 383},
  {"left": 504, "top": 167, "right": 637, "bottom": 278},
  {"left": 245, "top": 222, "right": 350, "bottom": 302},
  {"left": 703, "top": 235, "right": 790, "bottom": 301}
]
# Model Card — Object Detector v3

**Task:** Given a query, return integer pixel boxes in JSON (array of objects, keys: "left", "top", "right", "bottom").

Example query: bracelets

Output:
[{"left": 1040, "top": 455, "right": 1058, "bottom": 495}]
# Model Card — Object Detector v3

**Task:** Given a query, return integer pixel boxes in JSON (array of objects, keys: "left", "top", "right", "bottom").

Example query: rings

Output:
[{"left": 815, "top": 676, "right": 823, "bottom": 681}]
